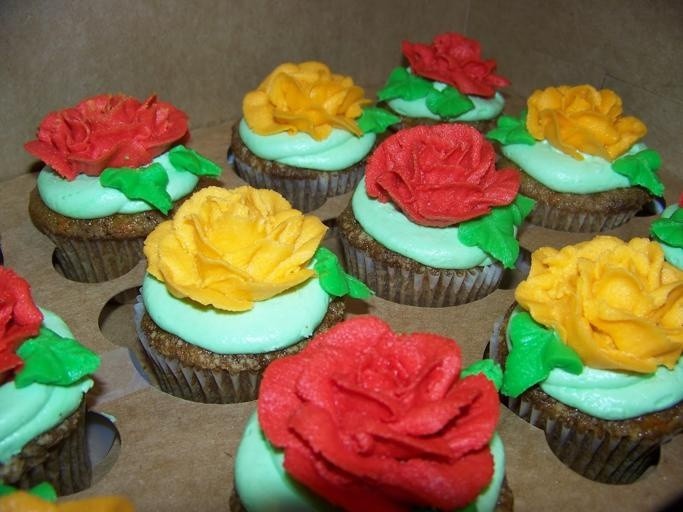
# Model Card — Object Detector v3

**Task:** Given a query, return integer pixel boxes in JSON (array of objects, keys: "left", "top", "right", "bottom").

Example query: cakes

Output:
[
  {"left": 337, "top": 123, "right": 537, "bottom": 307},
  {"left": 375, "top": 31, "right": 513, "bottom": 133},
  {"left": 134, "top": 183, "right": 375, "bottom": 404},
  {"left": 489, "top": 235, "right": 683, "bottom": 483},
  {"left": 482, "top": 84, "right": 666, "bottom": 266},
  {"left": 648, "top": 190, "right": 683, "bottom": 271},
  {"left": 25, "top": 93, "right": 225, "bottom": 301},
  {"left": 231, "top": 314, "right": 515, "bottom": 511},
  {"left": 0, "top": 264, "right": 102, "bottom": 496},
  {"left": 230, "top": 60, "right": 403, "bottom": 239},
  {"left": 1, "top": 482, "right": 137, "bottom": 512}
]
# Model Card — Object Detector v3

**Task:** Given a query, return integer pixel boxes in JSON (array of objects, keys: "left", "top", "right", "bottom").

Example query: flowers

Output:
[{"left": 1, "top": 31, "right": 683, "bottom": 511}]
